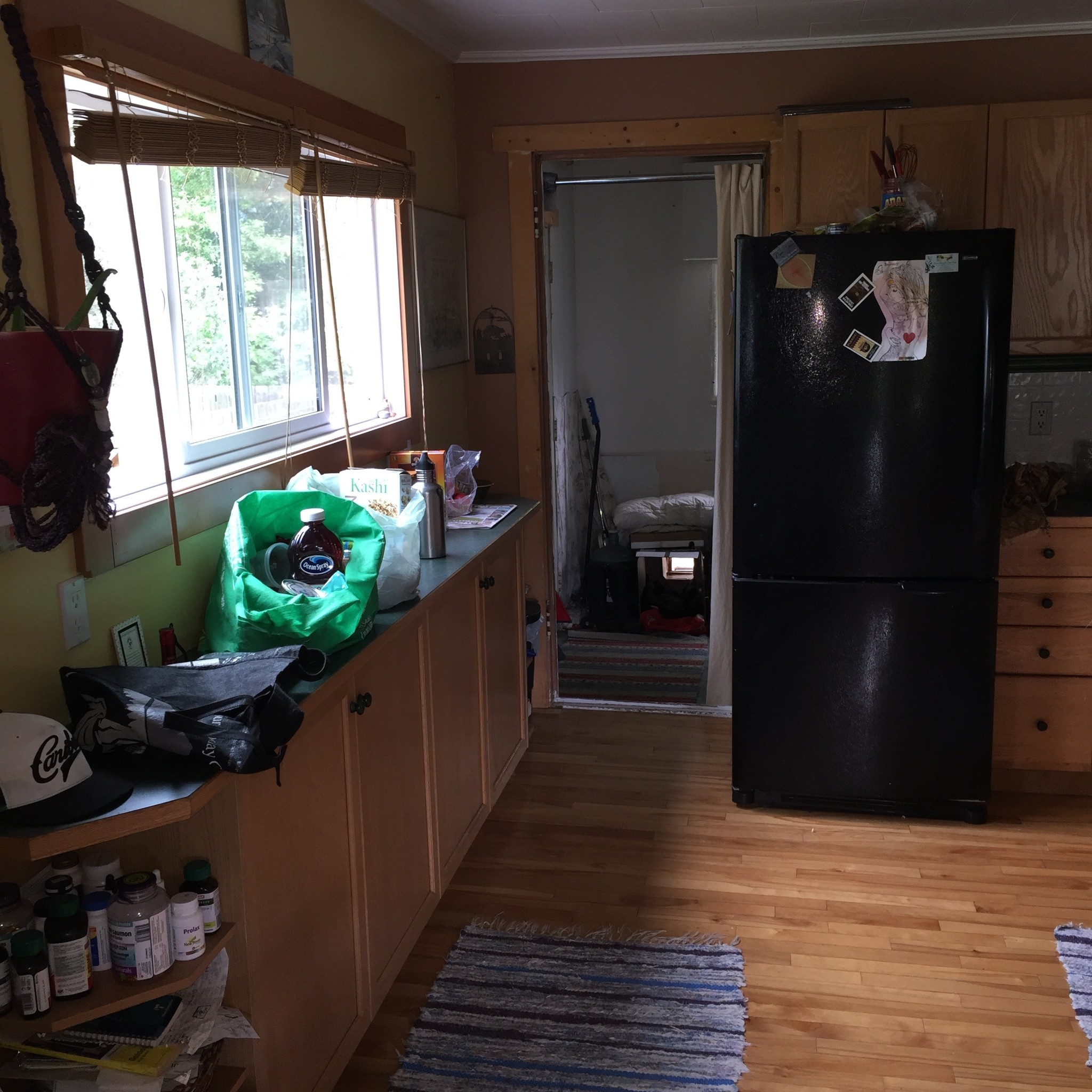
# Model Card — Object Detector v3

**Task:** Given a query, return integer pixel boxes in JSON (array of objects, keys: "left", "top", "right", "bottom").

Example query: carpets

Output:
[
  {"left": 1050, "top": 921, "right": 1092, "bottom": 1077},
  {"left": 391, "top": 910, "right": 753, "bottom": 1092}
]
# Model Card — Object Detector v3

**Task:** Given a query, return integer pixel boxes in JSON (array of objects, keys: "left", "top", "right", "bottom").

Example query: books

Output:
[
  {"left": 63, "top": 995, "right": 183, "bottom": 1048},
  {"left": 23, "top": 1031, "right": 183, "bottom": 1076}
]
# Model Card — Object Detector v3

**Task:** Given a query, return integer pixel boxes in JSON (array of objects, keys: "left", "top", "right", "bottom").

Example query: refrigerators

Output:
[{"left": 731, "top": 228, "right": 1016, "bottom": 826}]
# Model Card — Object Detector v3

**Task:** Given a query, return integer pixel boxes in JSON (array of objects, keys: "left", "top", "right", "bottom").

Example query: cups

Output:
[{"left": 249, "top": 542, "right": 293, "bottom": 589}]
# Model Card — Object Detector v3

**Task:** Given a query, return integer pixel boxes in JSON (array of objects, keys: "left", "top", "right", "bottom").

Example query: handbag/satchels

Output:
[
  {"left": 284, "top": 465, "right": 426, "bottom": 611},
  {"left": 60, "top": 644, "right": 327, "bottom": 774},
  {"left": 204, "top": 490, "right": 385, "bottom": 655}
]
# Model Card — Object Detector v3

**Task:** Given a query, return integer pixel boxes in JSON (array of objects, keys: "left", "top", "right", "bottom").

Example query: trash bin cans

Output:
[{"left": 525, "top": 598, "right": 542, "bottom": 703}]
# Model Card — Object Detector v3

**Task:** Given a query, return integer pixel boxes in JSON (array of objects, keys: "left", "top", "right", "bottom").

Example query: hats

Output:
[{"left": 0, "top": 711, "right": 133, "bottom": 831}]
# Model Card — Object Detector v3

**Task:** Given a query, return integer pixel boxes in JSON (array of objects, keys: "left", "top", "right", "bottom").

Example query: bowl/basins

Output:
[{"left": 472, "top": 480, "right": 494, "bottom": 504}]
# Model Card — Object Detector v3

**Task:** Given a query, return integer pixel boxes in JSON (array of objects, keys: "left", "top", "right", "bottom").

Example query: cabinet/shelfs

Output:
[
  {"left": 991, "top": 516, "right": 1092, "bottom": 797},
  {"left": 0, "top": 527, "right": 529, "bottom": 1092}
]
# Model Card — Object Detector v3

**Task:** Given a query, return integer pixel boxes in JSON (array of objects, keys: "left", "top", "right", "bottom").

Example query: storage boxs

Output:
[{"left": 390, "top": 450, "right": 446, "bottom": 501}]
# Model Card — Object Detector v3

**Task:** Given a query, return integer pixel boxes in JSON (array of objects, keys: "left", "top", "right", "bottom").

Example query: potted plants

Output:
[{"left": 0, "top": 268, "right": 122, "bottom": 507}]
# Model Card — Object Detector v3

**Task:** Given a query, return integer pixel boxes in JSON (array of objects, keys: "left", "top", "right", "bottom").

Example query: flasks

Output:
[{"left": 411, "top": 452, "right": 447, "bottom": 558}]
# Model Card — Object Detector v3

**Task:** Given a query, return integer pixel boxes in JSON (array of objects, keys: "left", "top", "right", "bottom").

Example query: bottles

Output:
[
  {"left": 288, "top": 508, "right": 344, "bottom": 590},
  {"left": 81, "top": 851, "right": 123, "bottom": 895},
  {"left": 45, "top": 874, "right": 78, "bottom": 896},
  {"left": 107, "top": 872, "right": 176, "bottom": 981},
  {"left": 171, "top": 892, "right": 206, "bottom": 961},
  {"left": 0, "top": 943, "right": 11, "bottom": 1017},
  {"left": 33, "top": 894, "right": 94, "bottom": 1000},
  {"left": 51, "top": 852, "right": 82, "bottom": 896},
  {"left": 152, "top": 869, "right": 166, "bottom": 892},
  {"left": 82, "top": 891, "right": 112, "bottom": 971},
  {"left": 10, "top": 930, "right": 51, "bottom": 1021},
  {"left": 179, "top": 860, "right": 221, "bottom": 936},
  {"left": 0, "top": 882, "right": 33, "bottom": 958},
  {"left": 880, "top": 178, "right": 913, "bottom": 229}
]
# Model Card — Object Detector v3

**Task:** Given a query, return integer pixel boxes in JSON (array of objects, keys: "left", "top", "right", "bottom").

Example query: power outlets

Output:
[
  {"left": 58, "top": 575, "right": 91, "bottom": 652},
  {"left": 1029, "top": 401, "right": 1053, "bottom": 436}
]
